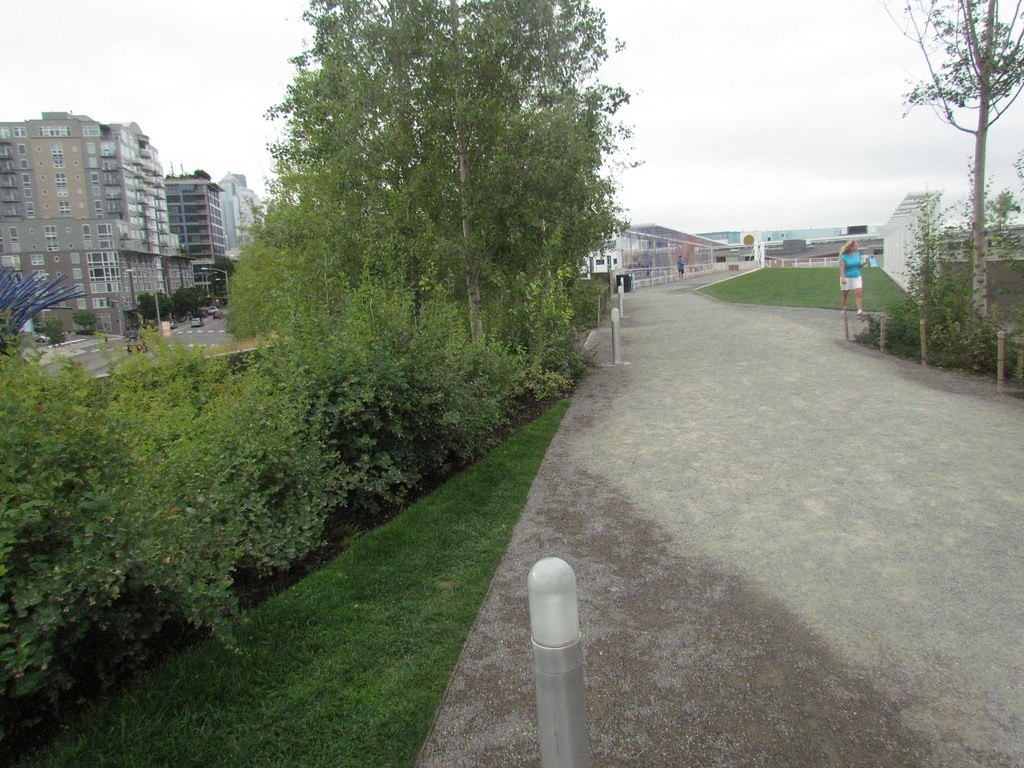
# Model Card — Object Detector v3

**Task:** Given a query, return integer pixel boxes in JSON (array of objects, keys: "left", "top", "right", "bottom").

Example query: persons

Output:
[
  {"left": 840, "top": 240, "right": 869, "bottom": 319},
  {"left": 675, "top": 256, "right": 686, "bottom": 281}
]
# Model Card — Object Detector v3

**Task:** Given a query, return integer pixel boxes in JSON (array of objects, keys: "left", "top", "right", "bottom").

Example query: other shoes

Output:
[
  {"left": 841, "top": 311, "right": 847, "bottom": 315},
  {"left": 857, "top": 309, "right": 863, "bottom": 314}
]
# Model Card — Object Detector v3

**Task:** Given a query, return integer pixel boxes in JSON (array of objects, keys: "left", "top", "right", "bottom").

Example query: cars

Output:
[
  {"left": 207, "top": 305, "right": 219, "bottom": 315},
  {"left": 213, "top": 310, "right": 225, "bottom": 319},
  {"left": 124, "top": 327, "right": 148, "bottom": 353},
  {"left": 190, "top": 318, "right": 204, "bottom": 328}
]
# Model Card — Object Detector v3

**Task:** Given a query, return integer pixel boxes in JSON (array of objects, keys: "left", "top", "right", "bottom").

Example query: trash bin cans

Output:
[
  {"left": 647, "top": 269, "right": 653, "bottom": 276},
  {"left": 616, "top": 274, "right": 632, "bottom": 292},
  {"left": 160, "top": 321, "right": 171, "bottom": 337}
]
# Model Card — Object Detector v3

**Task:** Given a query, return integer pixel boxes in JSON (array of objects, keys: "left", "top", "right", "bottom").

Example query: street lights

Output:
[
  {"left": 202, "top": 267, "right": 231, "bottom": 308},
  {"left": 205, "top": 271, "right": 218, "bottom": 298}
]
